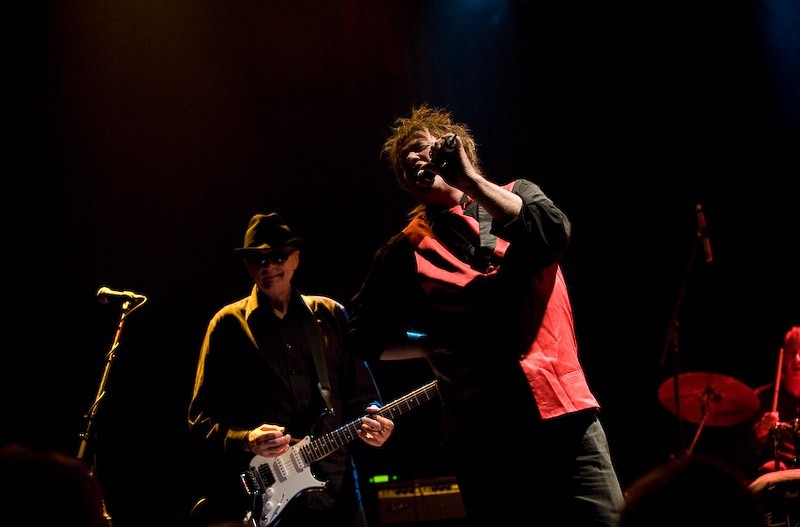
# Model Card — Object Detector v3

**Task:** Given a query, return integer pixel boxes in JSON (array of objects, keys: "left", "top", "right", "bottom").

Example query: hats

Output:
[{"left": 233, "top": 213, "right": 302, "bottom": 254}]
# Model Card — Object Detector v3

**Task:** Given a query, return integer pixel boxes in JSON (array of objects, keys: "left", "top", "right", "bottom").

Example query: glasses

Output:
[{"left": 247, "top": 249, "right": 295, "bottom": 266}]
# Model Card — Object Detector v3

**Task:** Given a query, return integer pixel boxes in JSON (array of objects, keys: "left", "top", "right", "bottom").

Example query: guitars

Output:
[{"left": 236, "top": 378, "right": 439, "bottom": 527}]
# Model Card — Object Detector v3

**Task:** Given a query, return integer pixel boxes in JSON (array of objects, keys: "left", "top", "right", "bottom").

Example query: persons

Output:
[
  {"left": 349, "top": 105, "right": 626, "bottom": 527},
  {"left": 0, "top": 369, "right": 113, "bottom": 527},
  {"left": 187, "top": 213, "right": 394, "bottom": 527},
  {"left": 736, "top": 326, "right": 800, "bottom": 527}
]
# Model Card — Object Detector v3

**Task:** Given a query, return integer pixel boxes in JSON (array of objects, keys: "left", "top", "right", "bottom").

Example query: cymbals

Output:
[{"left": 657, "top": 372, "right": 760, "bottom": 426}]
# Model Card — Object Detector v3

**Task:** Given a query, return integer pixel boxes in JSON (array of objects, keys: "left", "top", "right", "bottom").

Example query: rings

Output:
[{"left": 381, "top": 428, "right": 386, "bottom": 433}]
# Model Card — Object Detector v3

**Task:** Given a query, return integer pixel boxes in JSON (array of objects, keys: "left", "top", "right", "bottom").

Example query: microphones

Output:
[
  {"left": 691, "top": 196, "right": 715, "bottom": 265},
  {"left": 94, "top": 284, "right": 148, "bottom": 305},
  {"left": 416, "top": 131, "right": 458, "bottom": 187}
]
